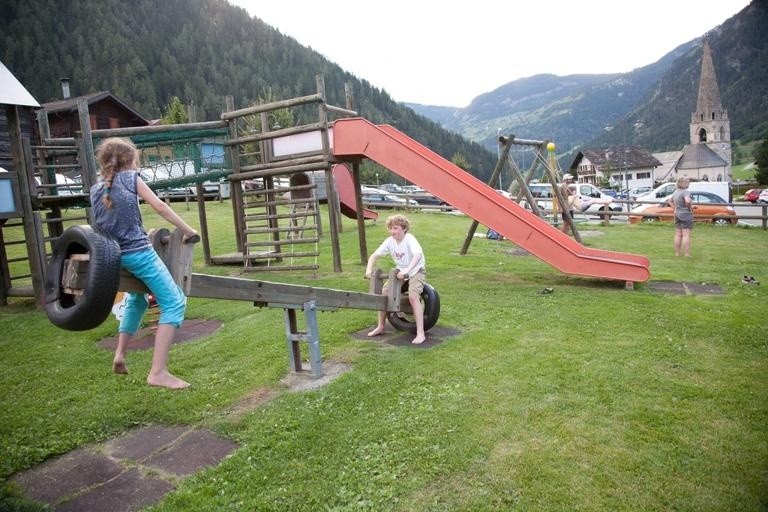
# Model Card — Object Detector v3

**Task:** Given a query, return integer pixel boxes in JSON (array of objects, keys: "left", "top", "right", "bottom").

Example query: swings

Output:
[{"left": 531, "top": 147, "right": 591, "bottom": 212}]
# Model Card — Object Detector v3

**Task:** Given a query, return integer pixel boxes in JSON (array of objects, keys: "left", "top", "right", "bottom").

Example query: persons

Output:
[
  {"left": 363, "top": 214, "right": 429, "bottom": 345},
  {"left": 88, "top": 134, "right": 197, "bottom": 391},
  {"left": 557, "top": 171, "right": 575, "bottom": 236},
  {"left": 667, "top": 175, "right": 693, "bottom": 258},
  {"left": 566, "top": 187, "right": 580, "bottom": 218}
]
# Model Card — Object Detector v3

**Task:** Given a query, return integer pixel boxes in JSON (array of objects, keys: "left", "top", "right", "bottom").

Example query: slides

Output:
[{"left": 332, "top": 117, "right": 651, "bottom": 283}]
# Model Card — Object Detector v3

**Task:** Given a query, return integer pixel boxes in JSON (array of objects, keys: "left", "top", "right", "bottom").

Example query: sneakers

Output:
[{"left": 742, "top": 276, "right": 759, "bottom": 286}]
